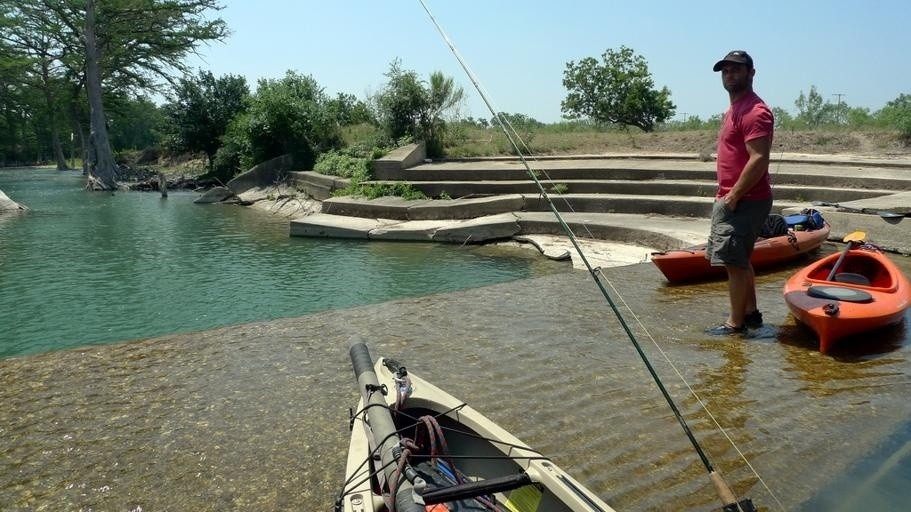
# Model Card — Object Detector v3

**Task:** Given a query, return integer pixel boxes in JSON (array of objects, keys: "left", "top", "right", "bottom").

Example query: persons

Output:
[{"left": 704, "top": 49, "right": 774, "bottom": 336}]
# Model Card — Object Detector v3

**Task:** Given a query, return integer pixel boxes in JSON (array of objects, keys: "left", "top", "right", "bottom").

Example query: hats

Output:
[{"left": 713, "top": 50, "right": 753, "bottom": 71}]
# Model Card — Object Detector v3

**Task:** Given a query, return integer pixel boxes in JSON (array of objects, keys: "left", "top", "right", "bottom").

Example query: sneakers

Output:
[
  {"left": 705, "top": 326, "right": 748, "bottom": 335},
  {"left": 743, "top": 309, "right": 764, "bottom": 326}
]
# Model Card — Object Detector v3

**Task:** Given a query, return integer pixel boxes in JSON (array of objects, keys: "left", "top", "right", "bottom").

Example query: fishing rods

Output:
[{"left": 420, "top": 1, "right": 754, "bottom": 511}]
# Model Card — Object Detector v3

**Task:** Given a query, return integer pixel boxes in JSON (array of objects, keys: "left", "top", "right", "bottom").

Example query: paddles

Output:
[{"left": 826, "top": 231, "right": 865, "bottom": 281}]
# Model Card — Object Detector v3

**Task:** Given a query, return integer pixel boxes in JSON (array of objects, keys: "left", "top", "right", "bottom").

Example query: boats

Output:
[
  {"left": 650, "top": 209, "right": 830, "bottom": 282},
  {"left": 783, "top": 243, "right": 911, "bottom": 354},
  {"left": 342, "top": 355, "right": 617, "bottom": 512}
]
